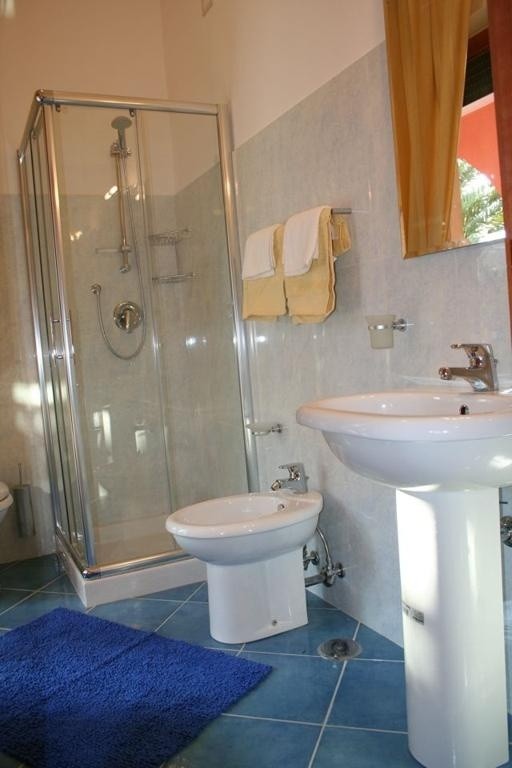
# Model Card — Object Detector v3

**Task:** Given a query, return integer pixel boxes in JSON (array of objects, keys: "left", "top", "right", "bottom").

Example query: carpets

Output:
[{"left": 0, "top": 607, "right": 274, "bottom": 768}]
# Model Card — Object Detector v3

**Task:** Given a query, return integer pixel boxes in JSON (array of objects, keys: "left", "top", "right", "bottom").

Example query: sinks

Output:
[
  {"left": 166, "top": 491, "right": 323, "bottom": 568},
  {"left": 297, "top": 386, "right": 512, "bottom": 487}
]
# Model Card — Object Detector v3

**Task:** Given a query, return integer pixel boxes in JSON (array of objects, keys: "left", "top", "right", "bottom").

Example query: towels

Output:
[{"left": 241, "top": 206, "right": 351, "bottom": 324}]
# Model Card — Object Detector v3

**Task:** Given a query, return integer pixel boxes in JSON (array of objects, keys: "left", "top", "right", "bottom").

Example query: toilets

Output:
[{"left": 0, "top": 480, "right": 15, "bottom": 523}]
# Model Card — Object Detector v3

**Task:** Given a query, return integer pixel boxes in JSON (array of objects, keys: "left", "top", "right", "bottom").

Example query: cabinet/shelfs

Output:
[{"left": 148, "top": 231, "right": 190, "bottom": 286}]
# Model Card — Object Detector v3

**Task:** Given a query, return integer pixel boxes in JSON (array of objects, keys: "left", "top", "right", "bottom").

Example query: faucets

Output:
[
  {"left": 439, "top": 342, "right": 497, "bottom": 392},
  {"left": 271, "top": 464, "right": 307, "bottom": 494}
]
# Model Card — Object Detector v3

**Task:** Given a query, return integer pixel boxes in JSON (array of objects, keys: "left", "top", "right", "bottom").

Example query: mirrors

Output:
[{"left": 385, "top": 0, "right": 512, "bottom": 259}]
